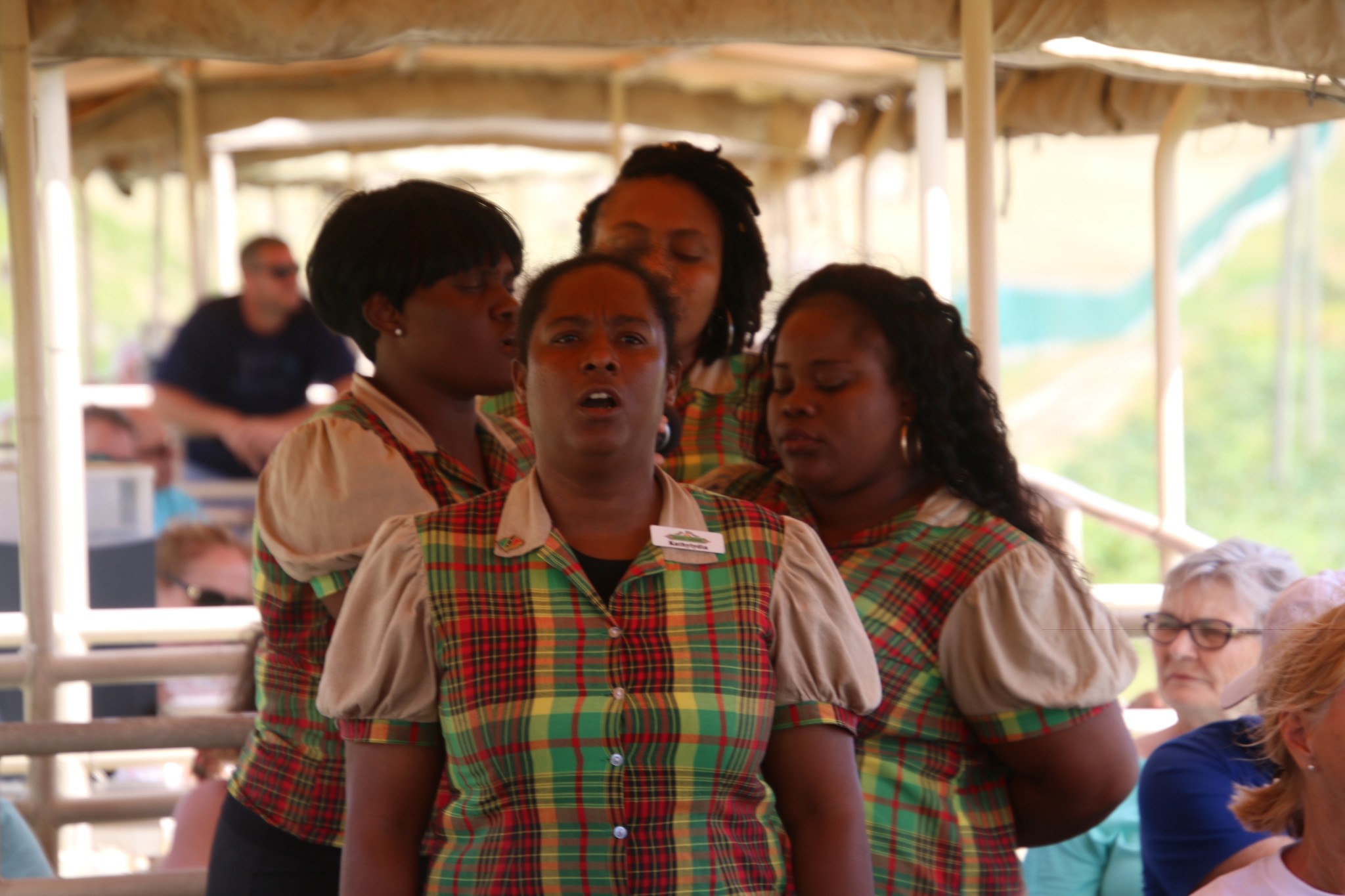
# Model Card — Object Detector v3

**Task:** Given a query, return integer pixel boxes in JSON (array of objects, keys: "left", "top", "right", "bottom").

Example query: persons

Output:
[
  {"left": 1188, "top": 604, "right": 1345, "bottom": 896},
  {"left": 201, "top": 178, "right": 536, "bottom": 895},
  {"left": 85, "top": 237, "right": 358, "bottom": 871},
  {"left": 1139, "top": 569, "right": 1345, "bottom": 896},
  {"left": 475, "top": 143, "right": 773, "bottom": 502},
  {"left": 315, "top": 256, "right": 885, "bottom": 895},
  {"left": 1018, "top": 539, "right": 1304, "bottom": 896},
  {"left": 684, "top": 262, "right": 1139, "bottom": 896}
]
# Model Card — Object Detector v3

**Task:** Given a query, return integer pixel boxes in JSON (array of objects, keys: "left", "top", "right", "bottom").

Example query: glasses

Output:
[
  {"left": 1143, "top": 612, "right": 1263, "bottom": 650},
  {"left": 256, "top": 264, "right": 299, "bottom": 278},
  {"left": 160, "top": 574, "right": 252, "bottom": 606}
]
